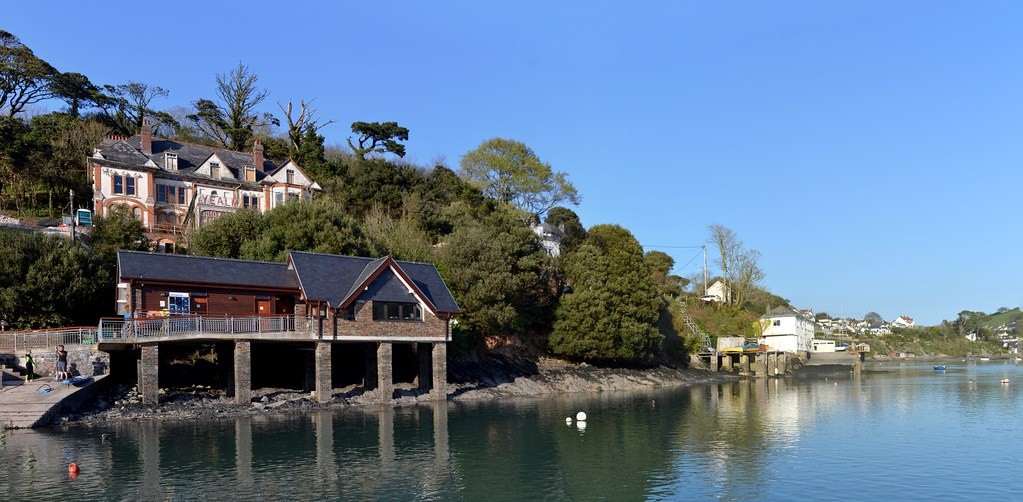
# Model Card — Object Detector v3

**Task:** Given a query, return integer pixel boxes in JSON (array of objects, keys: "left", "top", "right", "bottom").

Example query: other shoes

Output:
[
  {"left": 25, "top": 380, "right": 28, "bottom": 382},
  {"left": 59, "top": 378, "right": 63, "bottom": 380},
  {"left": 31, "top": 380, "right": 34, "bottom": 381}
]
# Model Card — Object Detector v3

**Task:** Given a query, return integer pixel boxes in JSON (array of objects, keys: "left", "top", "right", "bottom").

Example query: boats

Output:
[
  {"left": 933, "top": 365, "right": 946, "bottom": 371},
  {"left": 60, "top": 375, "right": 94, "bottom": 386}
]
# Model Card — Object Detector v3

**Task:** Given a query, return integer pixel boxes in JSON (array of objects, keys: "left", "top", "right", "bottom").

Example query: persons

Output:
[
  {"left": 124, "top": 304, "right": 131, "bottom": 330},
  {"left": 26, "top": 349, "right": 34, "bottom": 383},
  {"left": 56, "top": 345, "right": 68, "bottom": 381}
]
